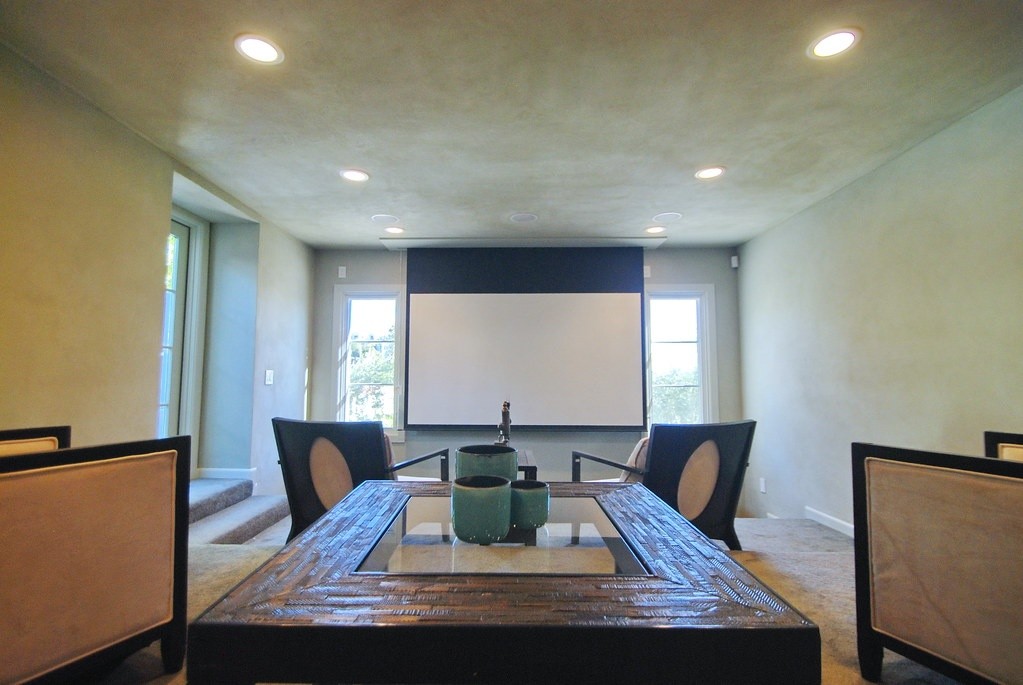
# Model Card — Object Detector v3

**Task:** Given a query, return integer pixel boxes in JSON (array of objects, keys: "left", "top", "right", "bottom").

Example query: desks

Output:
[{"left": 184, "top": 479, "right": 823, "bottom": 685}]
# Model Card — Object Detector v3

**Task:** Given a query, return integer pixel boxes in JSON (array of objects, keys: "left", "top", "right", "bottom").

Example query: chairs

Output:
[
  {"left": 572, "top": 418, "right": 756, "bottom": 553},
  {"left": 271, "top": 415, "right": 397, "bottom": 546}
]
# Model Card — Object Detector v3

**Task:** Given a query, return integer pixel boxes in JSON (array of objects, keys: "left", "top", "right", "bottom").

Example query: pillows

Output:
[{"left": 618, "top": 435, "right": 652, "bottom": 484}]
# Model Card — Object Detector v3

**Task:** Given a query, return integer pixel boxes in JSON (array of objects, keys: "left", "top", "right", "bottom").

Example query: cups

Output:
[
  {"left": 455, "top": 444, "right": 518, "bottom": 480},
  {"left": 451, "top": 475, "right": 511, "bottom": 544},
  {"left": 511, "top": 480, "right": 551, "bottom": 530}
]
